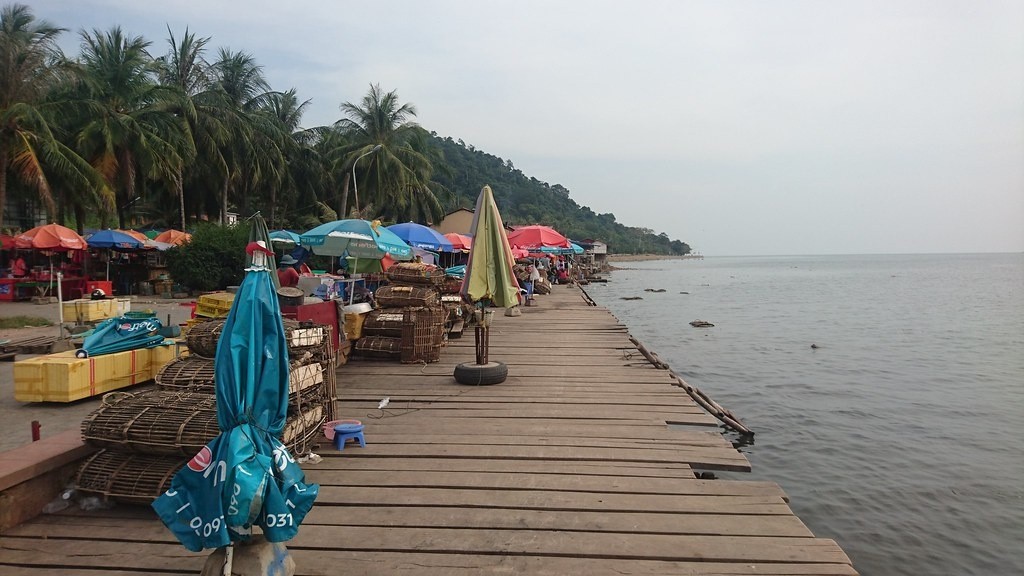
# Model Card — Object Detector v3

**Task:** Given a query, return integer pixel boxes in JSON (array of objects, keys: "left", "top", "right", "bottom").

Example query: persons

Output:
[
  {"left": 276, "top": 255, "right": 298, "bottom": 288},
  {"left": 546, "top": 264, "right": 571, "bottom": 284}
]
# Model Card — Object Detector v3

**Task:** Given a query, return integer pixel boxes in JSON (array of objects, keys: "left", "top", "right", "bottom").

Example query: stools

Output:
[{"left": 333, "top": 423, "right": 365, "bottom": 451}]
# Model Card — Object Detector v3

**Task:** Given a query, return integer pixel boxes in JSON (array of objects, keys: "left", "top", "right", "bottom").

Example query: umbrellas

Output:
[
  {"left": 444, "top": 220, "right": 583, "bottom": 262},
  {"left": 151, "top": 240, "right": 319, "bottom": 576},
  {"left": 268, "top": 229, "right": 302, "bottom": 260},
  {"left": 1, "top": 221, "right": 192, "bottom": 299},
  {"left": 458, "top": 183, "right": 524, "bottom": 364},
  {"left": 75, "top": 314, "right": 177, "bottom": 359},
  {"left": 383, "top": 220, "right": 453, "bottom": 256},
  {"left": 299, "top": 217, "right": 409, "bottom": 308}
]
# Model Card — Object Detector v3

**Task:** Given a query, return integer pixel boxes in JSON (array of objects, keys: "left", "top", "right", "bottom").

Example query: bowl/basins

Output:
[{"left": 324, "top": 420, "right": 361, "bottom": 440}]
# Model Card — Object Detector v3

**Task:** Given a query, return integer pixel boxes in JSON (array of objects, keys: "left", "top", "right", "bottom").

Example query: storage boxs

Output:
[{"left": 12, "top": 280, "right": 249, "bottom": 402}]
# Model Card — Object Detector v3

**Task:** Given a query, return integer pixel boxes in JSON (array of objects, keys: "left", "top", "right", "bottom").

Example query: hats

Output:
[{"left": 280, "top": 255, "right": 298, "bottom": 265}]
